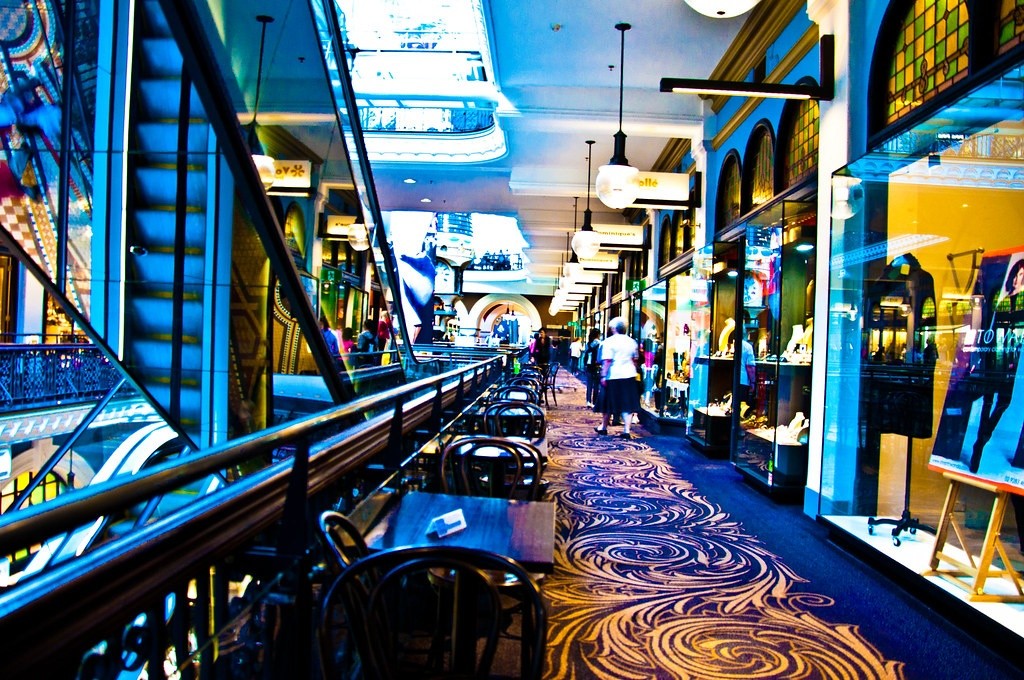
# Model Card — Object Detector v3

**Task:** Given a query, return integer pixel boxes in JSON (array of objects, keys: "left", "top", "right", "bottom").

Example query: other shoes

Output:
[
  {"left": 620, "top": 433, "right": 630, "bottom": 439},
  {"left": 970, "top": 445, "right": 982, "bottom": 473},
  {"left": 587, "top": 404, "right": 595, "bottom": 409},
  {"left": 594, "top": 427, "right": 607, "bottom": 435}
]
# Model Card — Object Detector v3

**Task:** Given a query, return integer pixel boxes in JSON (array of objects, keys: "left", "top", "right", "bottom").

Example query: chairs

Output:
[
  {"left": 437, "top": 362, "right": 564, "bottom": 501},
  {"left": 421, "top": 437, "right": 551, "bottom": 680},
  {"left": 314, "top": 510, "right": 370, "bottom": 576},
  {"left": 318, "top": 544, "right": 546, "bottom": 680}
]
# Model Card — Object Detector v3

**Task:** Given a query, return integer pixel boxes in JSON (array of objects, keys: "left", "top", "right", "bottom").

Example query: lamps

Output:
[
  {"left": 596, "top": 24, "right": 642, "bottom": 210},
  {"left": 548, "top": 171, "right": 702, "bottom": 317},
  {"left": 267, "top": 157, "right": 372, "bottom": 252},
  {"left": 242, "top": 15, "right": 276, "bottom": 193},
  {"left": 571, "top": 141, "right": 602, "bottom": 260},
  {"left": 660, "top": 34, "right": 836, "bottom": 101},
  {"left": 682, "top": 0, "right": 762, "bottom": 19}
]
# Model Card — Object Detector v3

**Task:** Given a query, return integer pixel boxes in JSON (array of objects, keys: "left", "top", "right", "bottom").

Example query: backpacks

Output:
[{"left": 584, "top": 342, "right": 599, "bottom": 365}]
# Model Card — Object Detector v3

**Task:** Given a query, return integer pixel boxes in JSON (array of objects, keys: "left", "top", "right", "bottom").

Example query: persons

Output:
[
  {"left": 318, "top": 310, "right": 391, "bottom": 369},
  {"left": 640, "top": 321, "right": 666, "bottom": 413},
  {"left": 863, "top": 252, "right": 939, "bottom": 439},
  {"left": 739, "top": 325, "right": 757, "bottom": 458},
  {"left": 467, "top": 249, "right": 511, "bottom": 271},
  {"left": 485, "top": 327, "right": 644, "bottom": 427},
  {"left": 591, "top": 317, "right": 639, "bottom": 440},
  {"left": 871, "top": 334, "right": 937, "bottom": 366},
  {"left": 967, "top": 259, "right": 1024, "bottom": 475}
]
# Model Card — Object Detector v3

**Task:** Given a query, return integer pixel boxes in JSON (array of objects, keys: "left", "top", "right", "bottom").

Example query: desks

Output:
[
  {"left": 476, "top": 383, "right": 535, "bottom": 401},
  {"left": 462, "top": 400, "right": 547, "bottom": 436},
  {"left": 360, "top": 489, "right": 557, "bottom": 577}
]
